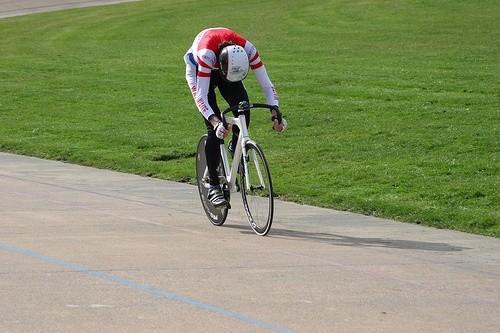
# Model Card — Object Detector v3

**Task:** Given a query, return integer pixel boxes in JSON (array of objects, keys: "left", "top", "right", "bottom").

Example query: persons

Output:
[{"left": 183, "top": 28, "right": 283, "bottom": 207}]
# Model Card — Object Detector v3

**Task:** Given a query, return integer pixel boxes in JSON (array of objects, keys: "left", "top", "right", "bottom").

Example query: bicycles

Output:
[{"left": 193, "top": 101, "right": 282, "bottom": 237}]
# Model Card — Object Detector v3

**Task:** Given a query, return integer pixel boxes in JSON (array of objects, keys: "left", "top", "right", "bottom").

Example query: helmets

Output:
[{"left": 219, "top": 45, "right": 249, "bottom": 82}]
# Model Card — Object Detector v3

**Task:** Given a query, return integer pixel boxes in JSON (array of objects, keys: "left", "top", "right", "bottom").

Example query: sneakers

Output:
[
  {"left": 207, "top": 189, "right": 228, "bottom": 208},
  {"left": 227, "top": 139, "right": 249, "bottom": 174}
]
